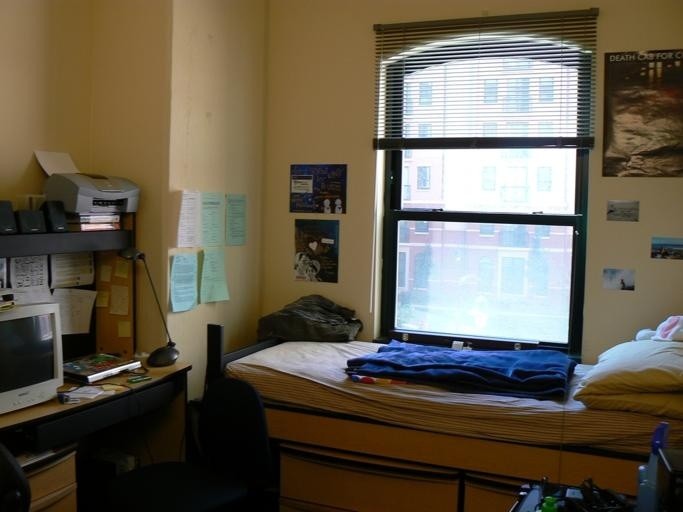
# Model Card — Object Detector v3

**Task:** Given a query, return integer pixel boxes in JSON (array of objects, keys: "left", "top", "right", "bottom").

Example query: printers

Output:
[{"left": 46, "top": 174, "right": 139, "bottom": 213}]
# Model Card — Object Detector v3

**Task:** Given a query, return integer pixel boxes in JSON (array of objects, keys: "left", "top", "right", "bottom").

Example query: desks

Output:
[{"left": 0, "top": 217, "right": 194, "bottom": 512}]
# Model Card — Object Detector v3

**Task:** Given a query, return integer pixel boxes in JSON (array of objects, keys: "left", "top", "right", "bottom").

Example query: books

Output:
[
  {"left": 66, "top": 215, "right": 120, "bottom": 223},
  {"left": 65, "top": 223, "right": 120, "bottom": 232}
]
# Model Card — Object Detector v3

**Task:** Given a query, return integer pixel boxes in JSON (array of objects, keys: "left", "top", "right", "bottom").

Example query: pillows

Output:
[{"left": 572, "top": 339, "right": 682, "bottom": 419}]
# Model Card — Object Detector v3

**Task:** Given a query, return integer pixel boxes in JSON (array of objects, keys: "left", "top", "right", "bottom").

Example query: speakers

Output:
[
  {"left": 15, "top": 211, "right": 45, "bottom": 234},
  {"left": 39, "top": 201, "right": 67, "bottom": 232},
  {"left": 0, "top": 201, "right": 16, "bottom": 233}
]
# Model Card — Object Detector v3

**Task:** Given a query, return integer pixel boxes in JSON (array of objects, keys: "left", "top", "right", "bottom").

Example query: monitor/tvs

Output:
[{"left": 1, "top": 303, "right": 63, "bottom": 415}]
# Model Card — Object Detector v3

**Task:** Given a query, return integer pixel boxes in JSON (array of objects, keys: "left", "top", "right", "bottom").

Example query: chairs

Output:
[{"left": 96, "top": 376, "right": 269, "bottom": 512}]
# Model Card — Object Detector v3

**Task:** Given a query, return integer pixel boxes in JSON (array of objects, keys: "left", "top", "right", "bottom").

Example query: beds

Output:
[{"left": 207, "top": 323, "right": 682, "bottom": 507}]
